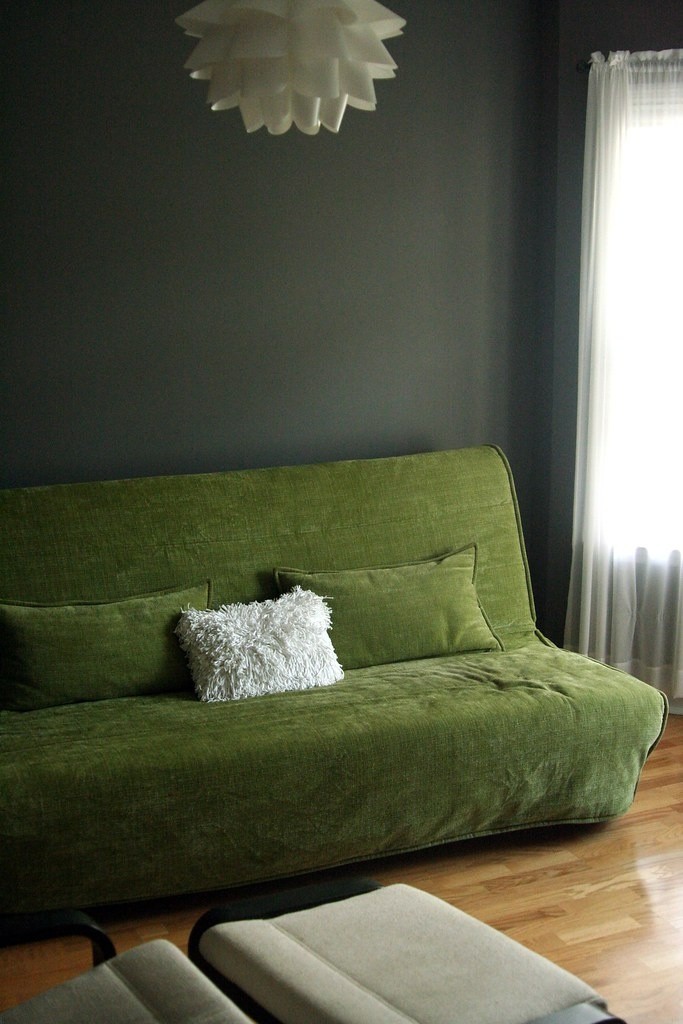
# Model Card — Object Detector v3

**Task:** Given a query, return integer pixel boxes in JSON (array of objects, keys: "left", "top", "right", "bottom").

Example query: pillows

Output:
[
  {"left": 2, "top": 577, "right": 213, "bottom": 710},
  {"left": 174, "top": 584, "right": 344, "bottom": 703},
  {"left": 274, "top": 542, "right": 506, "bottom": 672}
]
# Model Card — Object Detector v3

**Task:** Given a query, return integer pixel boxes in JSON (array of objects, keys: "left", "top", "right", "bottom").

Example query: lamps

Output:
[{"left": 174, "top": 0, "right": 408, "bottom": 135}]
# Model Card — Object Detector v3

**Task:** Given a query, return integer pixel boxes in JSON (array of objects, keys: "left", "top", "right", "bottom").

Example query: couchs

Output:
[{"left": 0, "top": 443, "right": 669, "bottom": 915}]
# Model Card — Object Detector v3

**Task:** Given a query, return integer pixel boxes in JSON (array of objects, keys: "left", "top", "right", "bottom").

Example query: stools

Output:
[{"left": 3, "top": 883, "right": 631, "bottom": 1022}]
